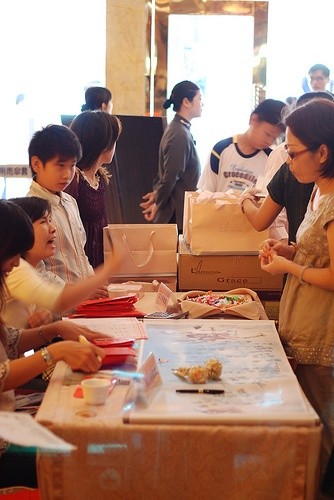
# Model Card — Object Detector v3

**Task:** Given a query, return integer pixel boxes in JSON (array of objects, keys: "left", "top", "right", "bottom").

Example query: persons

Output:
[
  {"left": 0, "top": 86, "right": 122, "bottom": 490},
  {"left": 199, "top": 99, "right": 291, "bottom": 193},
  {"left": 139, "top": 80, "right": 202, "bottom": 234},
  {"left": 243, "top": 64, "right": 334, "bottom": 485}
]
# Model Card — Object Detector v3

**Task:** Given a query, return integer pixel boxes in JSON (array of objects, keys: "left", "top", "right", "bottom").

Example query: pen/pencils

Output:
[
  {"left": 78, "top": 335, "right": 102, "bottom": 363},
  {"left": 83, "top": 375, "right": 130, "bottom": 385},
  {"left": 176, "top": 389, "right": 225, "bottom": 395}
]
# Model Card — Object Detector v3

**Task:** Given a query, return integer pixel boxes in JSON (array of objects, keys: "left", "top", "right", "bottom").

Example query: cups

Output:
[{"left": 81, "top": 379, "right": 111, "bottom": 405}]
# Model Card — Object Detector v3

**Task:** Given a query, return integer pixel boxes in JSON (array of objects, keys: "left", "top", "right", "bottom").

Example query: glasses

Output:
[{"left": 284, "top": 142, "right": 322, "bottom": 160}]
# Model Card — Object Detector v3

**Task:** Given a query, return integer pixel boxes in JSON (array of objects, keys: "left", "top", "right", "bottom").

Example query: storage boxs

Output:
[{"left": 177, "top": 234, "right": 284, "bottom": 292}]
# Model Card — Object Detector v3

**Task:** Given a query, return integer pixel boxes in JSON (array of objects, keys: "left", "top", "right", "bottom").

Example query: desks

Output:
[{"left": 34, "top": 319, "right": 324, "bottom": 500}]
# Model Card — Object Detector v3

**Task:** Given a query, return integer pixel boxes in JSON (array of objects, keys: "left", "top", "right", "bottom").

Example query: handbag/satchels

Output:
[
  {"left": 103, "top": 224, "right": 179, "bottom": 275},
  {"left": 183, "top": 188, "right": 269, "bottom": 256}
]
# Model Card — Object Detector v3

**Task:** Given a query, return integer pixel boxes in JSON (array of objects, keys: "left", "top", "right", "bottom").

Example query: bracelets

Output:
[
  {"left": 39, "top": 325, "right": 52, "bottom": 343},
  {"left": 240, "top": 198, "right": 255, "bottom": 214},
  {"left": 39, "top": 347, "right": 53, "bottom": 366},
  {"left": 298, "top": 263, "right": 314, "bottom": 287}
]
